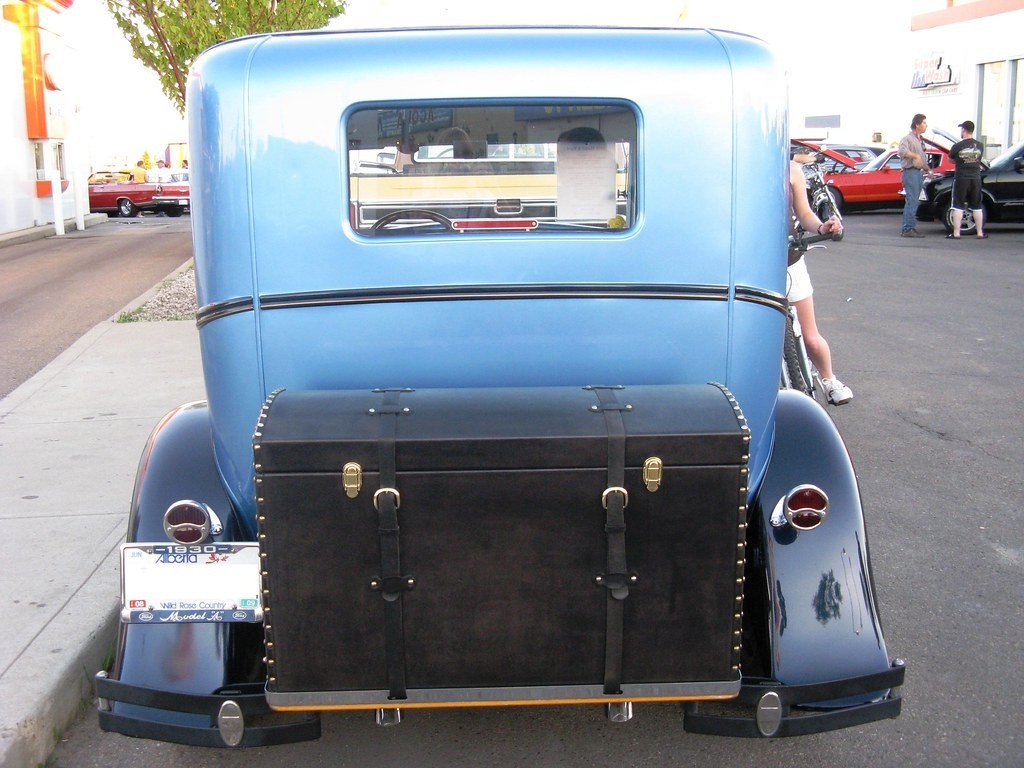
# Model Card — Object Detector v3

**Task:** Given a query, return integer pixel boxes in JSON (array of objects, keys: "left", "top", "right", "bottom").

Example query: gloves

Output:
[{"left": 813, "top": 154, "right": 825, "bottom": 164}]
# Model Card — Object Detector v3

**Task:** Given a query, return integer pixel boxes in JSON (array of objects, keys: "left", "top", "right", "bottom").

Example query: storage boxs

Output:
[{"left": 251, "top": 383, "right": 751, "bottom": 711}]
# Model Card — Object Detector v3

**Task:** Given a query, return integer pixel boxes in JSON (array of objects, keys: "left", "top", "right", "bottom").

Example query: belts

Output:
[{"left": 905, "top": 166, "right": 921, "bottom": 171}]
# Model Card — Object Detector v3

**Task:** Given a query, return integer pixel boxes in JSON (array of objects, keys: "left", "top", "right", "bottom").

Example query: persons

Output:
[
  {"left": 133, "top": 160, "right": 149, "bottom": 218},
  {"left": 945, "top": 121, "right": 989, "bottom": 239},
  {"left": 790, "top": 153, "right": 826, "bottom": 164},
  {"left": 898, "top": 114, "right": 931, "bottom": 238},
  {"left": 181, "top": 159, "right": 188, "bottom": 181},
  {"left": 786, "top": 160, "right": 854, "bottom": 406},
  {"left": 155, "top": 160, "right": 172, "bottom": 217}
]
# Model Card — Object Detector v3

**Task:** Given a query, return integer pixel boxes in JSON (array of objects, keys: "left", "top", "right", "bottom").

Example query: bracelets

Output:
[{"left": 817, "top": 223, "right": 823, "bottom": 236}]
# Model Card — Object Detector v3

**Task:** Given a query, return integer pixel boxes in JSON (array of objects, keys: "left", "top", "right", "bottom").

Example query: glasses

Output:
[{"left": 922, "top": 143, "right": 926, "bottom": 151}]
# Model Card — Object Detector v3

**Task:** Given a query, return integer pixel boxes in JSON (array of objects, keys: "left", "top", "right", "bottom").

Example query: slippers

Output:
[
  {"left": 977, "top": 234, "right": 989, "bottom": 239},
  {"left": 944, "top": 233, "right": 961, "bottom": 239}
]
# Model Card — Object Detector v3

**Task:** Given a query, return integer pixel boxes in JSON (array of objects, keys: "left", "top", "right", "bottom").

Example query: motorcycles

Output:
[{"left": 793, "top": 156, "right": 844, "bottom": 241}]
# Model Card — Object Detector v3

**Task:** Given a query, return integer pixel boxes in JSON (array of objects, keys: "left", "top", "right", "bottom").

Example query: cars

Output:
[
  {"left": 791, "top": 128, "right": 1024, "bottom": 235},
  {"left": 95, "top": 25, "right": 905, "bottom": 747},
  {"left": 88, "top": 168, "right": 190, "bottom": 218}
]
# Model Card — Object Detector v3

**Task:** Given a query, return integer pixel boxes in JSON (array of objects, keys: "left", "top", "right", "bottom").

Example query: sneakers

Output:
[
  {"left": 822, "top": 377, "right": 853, "bottom": 406},
  {"left": 901, "top": 228, "right": 925, "bottom": 238}
]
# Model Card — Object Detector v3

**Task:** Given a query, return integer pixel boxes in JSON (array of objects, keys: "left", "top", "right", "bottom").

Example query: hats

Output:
[{"left": 958, "top": 121, "right": 974, "bottom": 130}]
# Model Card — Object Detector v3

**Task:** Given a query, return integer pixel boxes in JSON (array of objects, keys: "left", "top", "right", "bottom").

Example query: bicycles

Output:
[{"left": 783, "top": 232, "right": 849, "bottom": 407}]
